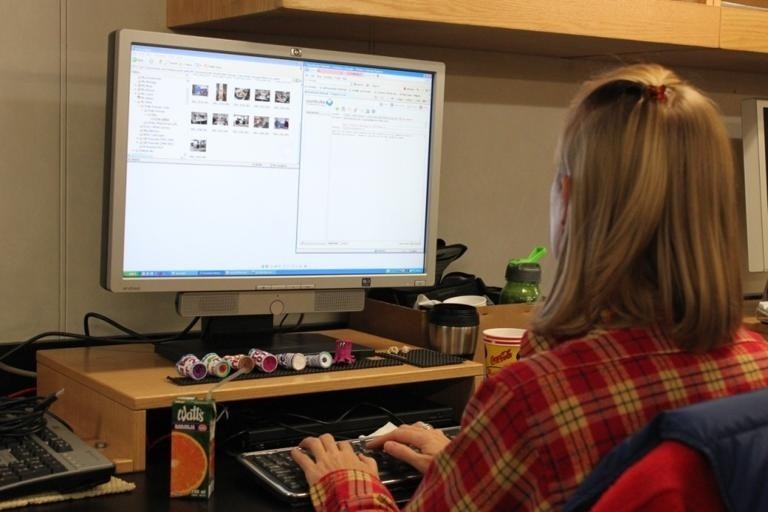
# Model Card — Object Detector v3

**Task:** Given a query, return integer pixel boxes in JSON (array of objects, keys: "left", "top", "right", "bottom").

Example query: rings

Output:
[{"left": 425, "top": 424, "right": 433, "bottom": 431}]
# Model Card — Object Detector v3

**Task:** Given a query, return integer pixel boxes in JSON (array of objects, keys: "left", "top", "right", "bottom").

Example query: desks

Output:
[{"left": 2, "top": 324, "right": 483, "bottom": 511}]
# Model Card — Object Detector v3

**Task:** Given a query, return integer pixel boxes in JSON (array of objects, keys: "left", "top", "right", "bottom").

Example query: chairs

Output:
[{"left": 556, "top": 392, "right": 766, "bottom": 511}]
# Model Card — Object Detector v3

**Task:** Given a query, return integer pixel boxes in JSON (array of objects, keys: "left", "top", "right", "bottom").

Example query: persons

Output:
[{"left": 291, "top": 60, "right": 768, "bottom": 512}]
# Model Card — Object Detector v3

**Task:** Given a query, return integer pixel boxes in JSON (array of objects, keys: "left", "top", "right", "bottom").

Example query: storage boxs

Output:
[{"left": 346, "top": 283, "right": 551, "bottom": 367}]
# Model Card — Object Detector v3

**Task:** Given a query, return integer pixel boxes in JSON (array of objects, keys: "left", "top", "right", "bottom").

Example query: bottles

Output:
[{"left": 497, "top": 258, "right": 543, "bottom": 302}]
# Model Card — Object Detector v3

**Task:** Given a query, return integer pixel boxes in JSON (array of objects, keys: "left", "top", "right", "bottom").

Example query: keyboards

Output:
[
  {"left": 0, "top": 408, "right": 116, "bottom": 500},
  {"left": 240, "top": 424, "right": 462, "bottom": 505}
]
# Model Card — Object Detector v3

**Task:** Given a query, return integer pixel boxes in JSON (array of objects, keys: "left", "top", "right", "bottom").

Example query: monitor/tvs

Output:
[
  {"left": 741, "top": 99, "right": 768, "bottom": 321},
  {"left": 100, "top": 27, "right": 447, "bottom": 364}
]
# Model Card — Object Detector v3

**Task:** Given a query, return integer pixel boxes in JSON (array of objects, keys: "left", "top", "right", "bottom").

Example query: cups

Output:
[
  {"left": 442, "top": 295, "right": 486, "bottom": 309},
  {"left": 429, "top": 303, "right": 480, "bottom": 361},
  {"left": 483, "top": 325, "right": 528, "bottom": 376}
]
